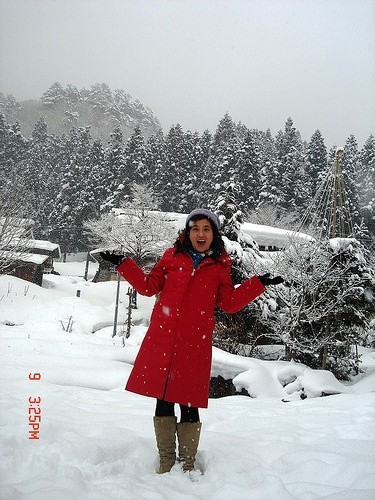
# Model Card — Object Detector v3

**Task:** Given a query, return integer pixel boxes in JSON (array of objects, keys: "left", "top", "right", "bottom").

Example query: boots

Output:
[
  {"left": 176, "top": 422, "right": 203, "bottom": 472},
  {"left": 153, "top": 416, "right": 177, "bottom": 474}
]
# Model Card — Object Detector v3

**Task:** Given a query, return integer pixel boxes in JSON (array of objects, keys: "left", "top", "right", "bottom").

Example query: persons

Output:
[{"left": 98, "top": 208, "right": 286, "bottom": 477}]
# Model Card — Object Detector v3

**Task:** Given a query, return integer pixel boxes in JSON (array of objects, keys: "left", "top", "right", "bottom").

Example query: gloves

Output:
[
  {"left": 99, "top": 250, "right": 126, "bottom": 266},
  {"left": 257, "top": 272, "right": 284, "bottom": 285}
]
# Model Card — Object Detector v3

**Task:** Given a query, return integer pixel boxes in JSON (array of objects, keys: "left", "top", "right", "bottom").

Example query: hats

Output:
[{"left": 185, "top": 208, "right": 220, "bottom": 231}]
